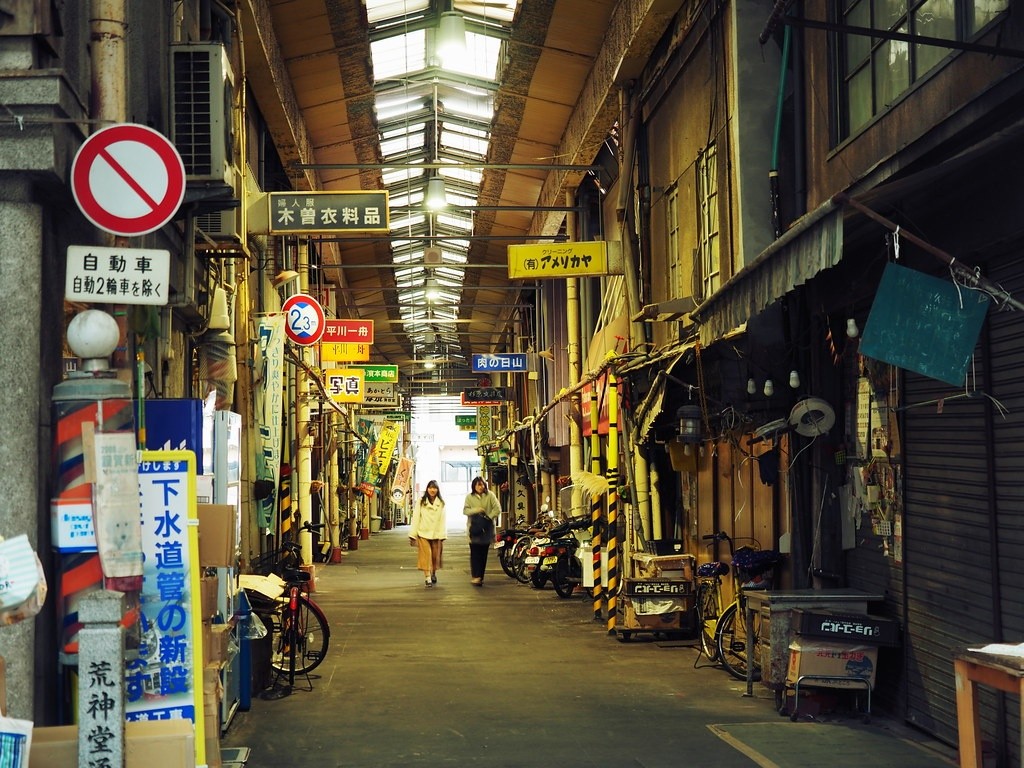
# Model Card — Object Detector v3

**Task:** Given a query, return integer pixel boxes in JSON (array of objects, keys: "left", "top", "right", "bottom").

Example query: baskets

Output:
[
  {"left": 251, "top": 548, "right": 291, "bottom": 579},
  {"left": 737, "top": 561, "right": 780, "bottom": 590}
]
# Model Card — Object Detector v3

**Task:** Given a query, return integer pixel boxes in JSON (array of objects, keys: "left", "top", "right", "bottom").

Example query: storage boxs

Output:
[
  {"left": 197, "top": 503, "right": 236, "bottom": 568},
  {"left": 132, "top": 397, "right": 204, "bottom": 476},
  {"left": 211, "top": 624, "right": 230, "bottom": 660},
  {"left": 200, "top": 576, "right": 218, "bottom": 621},
  {"left": 29, "top": 717, "right": 195, "bottom": 768},
  {"left": 791, "top": 606, "right": 897, "bottom": 647},
  {"left": 634, "top": 552, "right": 695, "bottom": 581},
  {"left": 623, "top": 596, "right": 680, "bottom": 628},
  {"left": 621, "top": 578, "right": 693, "bottom": 597},
  {"left": 787, "top": 636, "right": 878, "bottom": 689},
  {"left": 203, "top": 660, "right": 222, "bottom": 768},
  {"left": 202, "top": 620, "right": 212, "bottom": 670},
  {"left": 645, "top": 538, "right": 684, "bottom": 556}
]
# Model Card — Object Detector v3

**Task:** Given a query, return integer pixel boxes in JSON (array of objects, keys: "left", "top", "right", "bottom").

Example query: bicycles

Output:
[
  {"left": 583, "top": 510, "right": 624, "bottom": 599},
  {"left": 510, "top": 514, "right": 552, "bottom": 584},
  {"left": 339, "top": 509, "right": 362, "bottom": 545},
  {"left": 251, "top": 521, "right": 331, "bottom": 692},
  {"left": 692, "top": 530, "right": 776, "bottom": 681}
]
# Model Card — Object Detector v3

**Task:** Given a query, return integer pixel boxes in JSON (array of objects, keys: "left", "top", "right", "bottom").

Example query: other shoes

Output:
[
  {"left": 432, "top": 575, "right": 437, "bottom": 583},
  {"left": 425, "top": 576, "right": 432, "bottom": 586},
  {"left": 472, "top": 577, "right": 482, "bottom": 585}
]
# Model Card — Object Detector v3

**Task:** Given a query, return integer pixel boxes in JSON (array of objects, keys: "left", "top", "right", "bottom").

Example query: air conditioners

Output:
[
  {"left": 194, "top": 161, "right": 245, "bottom": 244},
  {"left": 170, "top": 40, "right": 232, "bottom": 189}
]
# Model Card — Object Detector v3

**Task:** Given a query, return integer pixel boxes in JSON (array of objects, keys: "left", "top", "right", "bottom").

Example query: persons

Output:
[
  {"left": 463, "top": 476, "right": 502, "bottom": 588},
  {"left": 408, "top": 480, "right": 448, "bottom": 588}
]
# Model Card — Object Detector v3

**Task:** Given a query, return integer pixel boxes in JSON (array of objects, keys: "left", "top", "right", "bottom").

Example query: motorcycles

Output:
[{"left": 494, "top": 495, "right": 592, "bottom": 598}]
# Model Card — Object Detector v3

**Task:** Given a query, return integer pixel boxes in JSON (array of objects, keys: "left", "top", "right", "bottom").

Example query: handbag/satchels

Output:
[{"left": 469, "top": 512, "right": 493, "bottom": 540}]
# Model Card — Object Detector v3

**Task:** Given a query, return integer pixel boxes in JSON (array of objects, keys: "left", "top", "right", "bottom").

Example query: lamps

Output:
[
  {"left": 248, "top": 258, "right": 300, "bottom": 289},
  {"left": 435, "top": 0, "right": 467, "bottom": 59},
  {"left": 539, "top": 343, "right": 569, "bottom": 360},
  {"left": 431, "top": 371, "right": 438, "bottom": 383},
  {"left": 426, "top": 77, "right": 447, "bottom": 207},
  {"left": 425, "top": 299, "right": 435, "bottom": 343},
  {"left": 424, "top": 354, "right": 435, "bottom": 368},
  {"left": 440, "top": 354, "right": 447, "bottom": 396},
  {"left": 425, "top": 213, "right": 440, "bottom": 299},
  {"left": 676, "top": 404, "right": 704, "bottom": 443}
]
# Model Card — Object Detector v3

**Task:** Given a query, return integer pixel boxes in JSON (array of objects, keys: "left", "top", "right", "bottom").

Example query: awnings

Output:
[{"left": 689, "top": 80, "right": 1024, "bottom": 352}]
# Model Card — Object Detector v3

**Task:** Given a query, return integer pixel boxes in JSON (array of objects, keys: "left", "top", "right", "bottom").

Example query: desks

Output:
[{"left": 951, "top": 650, "right": 1024, "bottom": 768}]
